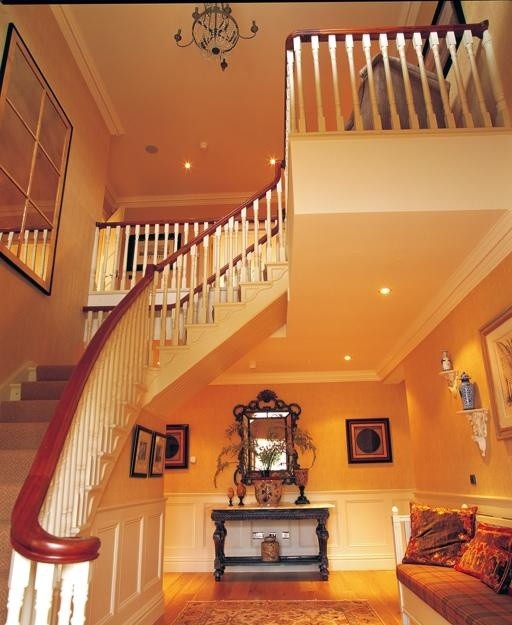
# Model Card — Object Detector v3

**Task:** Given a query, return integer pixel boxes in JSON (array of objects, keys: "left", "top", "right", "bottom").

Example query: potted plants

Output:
[{"left": 213, "top": 419, "right": 316, "bottom": 507}]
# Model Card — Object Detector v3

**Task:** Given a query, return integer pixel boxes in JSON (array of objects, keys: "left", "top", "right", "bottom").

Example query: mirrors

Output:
[{"left": 233, "top": 389, "right": 302, "bottom": 490}]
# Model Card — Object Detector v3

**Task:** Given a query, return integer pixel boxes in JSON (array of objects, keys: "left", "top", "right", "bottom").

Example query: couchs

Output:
[{"left": 390, "top": 504, "right": 512, "bottom": 625}]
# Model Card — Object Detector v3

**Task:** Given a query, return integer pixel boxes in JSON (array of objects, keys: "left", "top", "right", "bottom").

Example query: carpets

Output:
[{"left": 170, "top": 599, "right": 386, "bottom": 625}]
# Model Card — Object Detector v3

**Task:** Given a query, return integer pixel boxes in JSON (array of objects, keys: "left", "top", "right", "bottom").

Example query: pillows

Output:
[
  {"left": 402, "top": 502, "right": 479, "bottom": 566},
  {"left": 455, "top": 521, "right": 512, "bottom": 594}
]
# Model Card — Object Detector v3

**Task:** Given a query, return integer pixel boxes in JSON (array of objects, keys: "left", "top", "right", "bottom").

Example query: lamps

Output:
[{"left": 174, "top": 2, "right": 259, "bottom": 73}]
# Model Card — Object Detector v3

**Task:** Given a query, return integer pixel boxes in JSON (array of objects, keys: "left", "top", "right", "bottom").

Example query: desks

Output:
[{"left": 209, "top": 505, "right": 337, "bottom": 581}]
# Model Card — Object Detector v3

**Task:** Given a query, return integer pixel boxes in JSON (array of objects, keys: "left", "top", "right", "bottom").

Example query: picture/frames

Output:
[
  {"left": 346, "top": 418, "right": 393, "bottom": 464},
  {"left": 416, "top": 0, "right": 465, "bottom": 80},
  {"left": 165, "top": 423, "right": 189, "bottom": 470},
  {"left": 149, "top": 431, "right": 165, "bottom": 477},
  {"left": 478, "top": 303, "right": 512, "bottom": 441},
  {"left": 130, "top": 423, "right": 152, "bottom": 478},
  {"left": 126, "top": 233, "right": 181, "bottom": 272}
]
